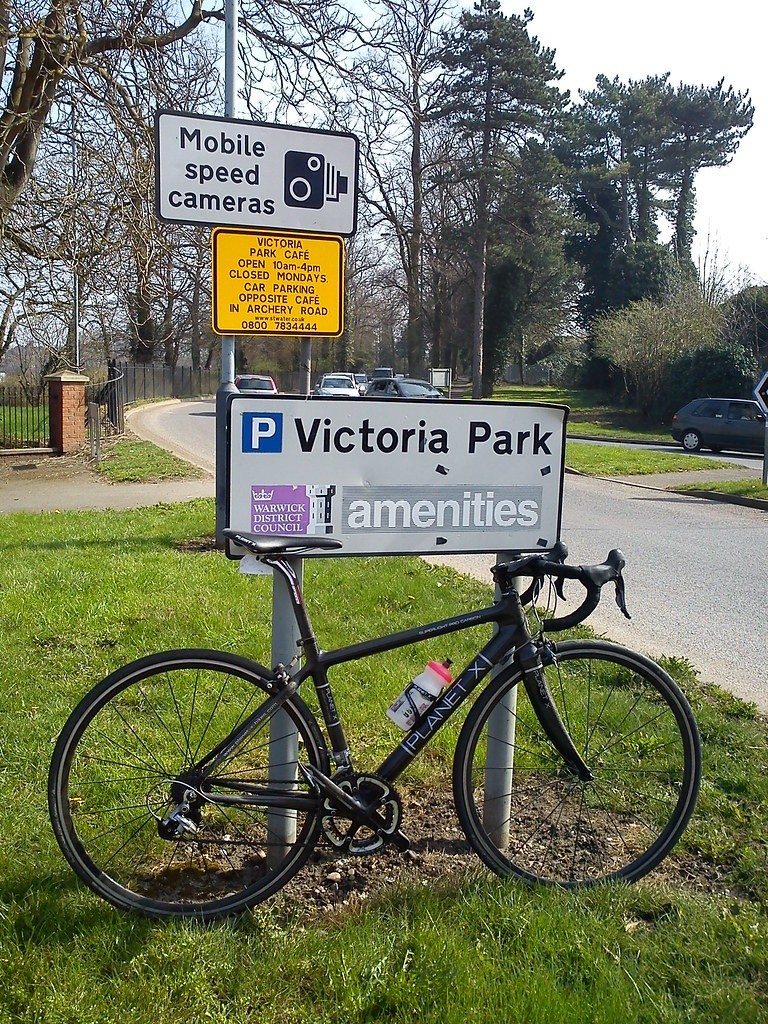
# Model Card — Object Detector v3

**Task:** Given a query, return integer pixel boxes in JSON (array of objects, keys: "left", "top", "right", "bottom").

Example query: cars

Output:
[
  {"left": 234, "top": 375, "right": 277, "bottom": 395},
  {"left": 313, "top": 375, "right": 360, "bottom": 397},
  {"left": 365, "top": 378, "right": 444, "bottom": 399},
  {"left": 325, "top": 367, "right": 404, "bottom": 395},
  {"left": 671, "top": 397, "right": 768, "bottom": 453}
]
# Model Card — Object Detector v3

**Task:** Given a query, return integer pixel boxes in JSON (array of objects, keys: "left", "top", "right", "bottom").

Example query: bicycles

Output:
[{"left": 47, "top": 528, "right": 703, "bottom": 922}]
